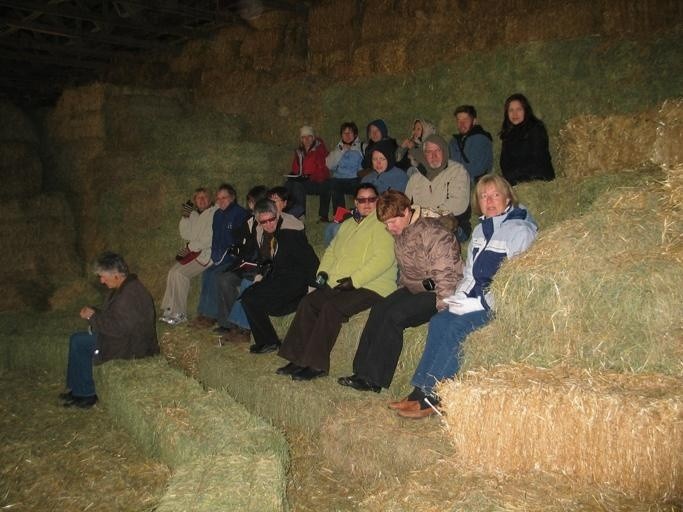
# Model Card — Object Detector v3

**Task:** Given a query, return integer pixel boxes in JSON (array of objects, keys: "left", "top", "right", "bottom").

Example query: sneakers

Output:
[
  {"left": 57, "top": 390, "right": 99, "bottom": 410},
  {"left": 316, "top": 216, "right": 330, "bottom": 224},
  {"left": 157, "top": 308, "right": 189, "bottom": 327},
  {"left": 190, "top": 315, "right": 252, "bottom": 344}
]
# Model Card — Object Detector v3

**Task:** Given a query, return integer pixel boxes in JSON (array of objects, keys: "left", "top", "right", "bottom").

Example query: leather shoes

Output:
[
  {"left": 275, "top": 361, "right": 329, "bottom": 382},
  {"left": 248, "top": 339, "right": 281, "bottom": 355},
  {"left": 337, "top": 375, "right": 382, "bottom": 393},
  {"left": 387, "top": 396, "right": 443, "bottom": 420}
]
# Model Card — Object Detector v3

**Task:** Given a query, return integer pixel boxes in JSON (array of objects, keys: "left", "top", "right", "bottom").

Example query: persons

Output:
[
  {"left": 498, "top": 95, "right": 555, "bottom": 188},
  {"left": 59, "top": 252, "right": 160, "bottom": 410},
  {"left": 393, "top": 104, "right": 492, "bottom": 240},
  {"left": 279, "top": 120, "right": 399, "bottom": 224},
  {"left": 272, "top": 183, "right": 400, "bottom": 382},
  {"left": 335, "top": 189, "right": 464, "bottom": 393},
  {"left": 386, "top": 174, "right": 540, "bottom": 419},
  {"left": 159, "top": 183, "right": 321, "bottom": 353}
]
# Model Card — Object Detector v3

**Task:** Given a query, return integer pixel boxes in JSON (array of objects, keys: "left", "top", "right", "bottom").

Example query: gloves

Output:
[
  {"left": 308, "top": 271, "right": 328, "bottom": 289},
  {"left": 332, "top": 276, "right": 355, "bottom": 291},
  {"left": 181, "top": 199, "right": 193, "bottom": 217},
  {"left": 441, "top": 290, "right": 486, "bottom": 316},
  {"left": 175, "top": 247, "right": 190, "bottom": 261}
]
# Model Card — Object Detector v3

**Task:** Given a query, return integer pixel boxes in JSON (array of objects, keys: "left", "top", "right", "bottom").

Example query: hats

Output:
[{"left": 299, "top": 125, "right": 315, "bottom": 140}]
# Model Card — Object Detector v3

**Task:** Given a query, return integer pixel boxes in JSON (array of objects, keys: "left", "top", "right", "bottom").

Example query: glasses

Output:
[
  {"left": 354, "top": 196, "right": 378, "bottom": 204},
  {"left": 257, "top": 213, "right": 277, "bottom": 225}
]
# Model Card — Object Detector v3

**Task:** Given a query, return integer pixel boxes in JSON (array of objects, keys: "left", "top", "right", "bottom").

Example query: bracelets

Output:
[{"left": 87, "top": 312, "right": 93, "bottom": 320}]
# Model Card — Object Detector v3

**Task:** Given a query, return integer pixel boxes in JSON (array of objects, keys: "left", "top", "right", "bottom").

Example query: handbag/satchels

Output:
[{"left": 176, "top": 249, "right": 201, "bottom": 266}]
